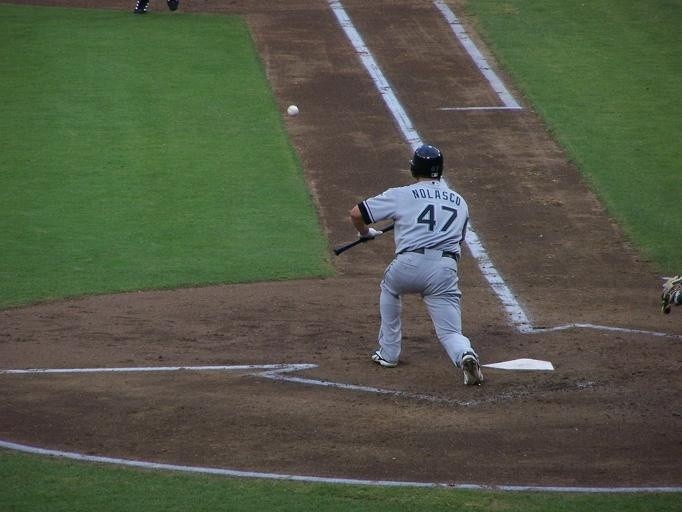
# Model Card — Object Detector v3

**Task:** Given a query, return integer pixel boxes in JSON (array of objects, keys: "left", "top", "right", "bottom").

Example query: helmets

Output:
[{"left": 411, "top": 145, "right": 443, "bottom": 179}]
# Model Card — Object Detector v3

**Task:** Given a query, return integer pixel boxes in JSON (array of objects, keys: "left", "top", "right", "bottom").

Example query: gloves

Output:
[{"left": 358, "top": 228, "right": 383, "bottom": 240}]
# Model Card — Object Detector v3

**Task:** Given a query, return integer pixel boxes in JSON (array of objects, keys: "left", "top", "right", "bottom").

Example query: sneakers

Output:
[
  {"left": 134, "top": 0, "right": 150, "bottom": 13},
  {"left": 370, "top": 351, "right": 398, "bottom": 368},
  {"left": 460, "top": 353, "right": 484, "bottom": 386},
  {"left": 660, "top": 289, "right": 672, "bottom": 315},
  {"left": 168, "top": 0, "right": 179, "bottom": 11}
]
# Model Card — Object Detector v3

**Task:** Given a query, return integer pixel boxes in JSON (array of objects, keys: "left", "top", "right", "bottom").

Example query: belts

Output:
[{"left": 406, "top": 247, "right": 458, "bottom": 262}]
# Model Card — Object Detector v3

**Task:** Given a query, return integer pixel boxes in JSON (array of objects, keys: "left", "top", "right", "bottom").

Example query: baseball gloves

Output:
[{"left": 661, "top": 275, "right": 681, "bottom": 314}]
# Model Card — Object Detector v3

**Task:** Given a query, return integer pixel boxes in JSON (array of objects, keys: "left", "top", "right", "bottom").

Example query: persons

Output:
[
  {"left": 133, "top": 1, "right": 180, "bottom": 14},
  {"left": 351, "top": 145, "right": 484, "bottom": 386}
]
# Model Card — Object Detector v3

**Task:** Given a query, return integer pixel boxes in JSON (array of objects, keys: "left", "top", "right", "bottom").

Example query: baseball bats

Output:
[{"left": 334, "top": 223, "right": 394, "bottom": 256}]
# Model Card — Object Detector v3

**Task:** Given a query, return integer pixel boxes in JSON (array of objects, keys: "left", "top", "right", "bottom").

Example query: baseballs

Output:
[{"left": 287, "top": 105, "right": 299, "bottom": 116}]
